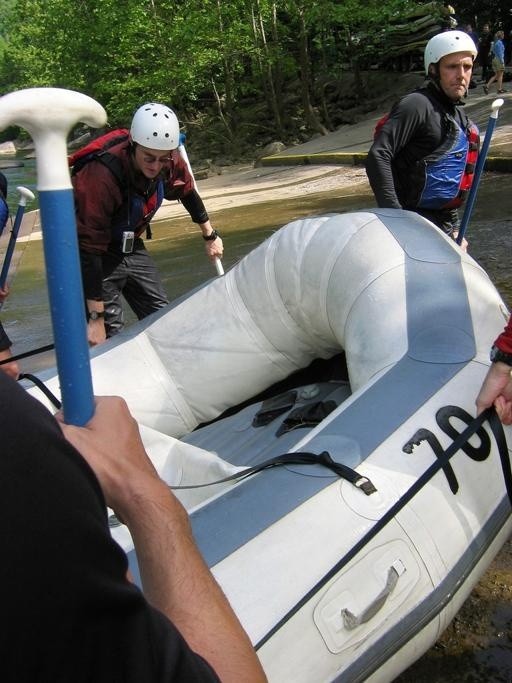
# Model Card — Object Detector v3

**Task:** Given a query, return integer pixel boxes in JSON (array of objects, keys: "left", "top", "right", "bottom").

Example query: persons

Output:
[
  {"left": 68, "top": 100, "right": 226, "bottom": 347},
  {"left": 0, "top": 317, "right": 20, "bottom": 382},
  {"left": 0, "top": 279, "right": 10, "bottom": 303},
  {"left": 364, "top": 28, "right": 481, "bottom": 253},
  {"left": 440, "top": 14, "right": 512, "bottom": 94},
  {"left": 0, "top": 361, "right": 270, "bottom": 681},
  {"left": 476, "top": 316, "right": 511, "bottom": 425}
]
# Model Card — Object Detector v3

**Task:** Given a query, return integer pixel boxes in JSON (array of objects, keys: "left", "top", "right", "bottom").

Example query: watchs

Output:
[
  {"left": 85, "top": 310, "right": 106, "bottom": 320},
  {"left": 201, "top": 228, "right": 219, "bottom": 242}
]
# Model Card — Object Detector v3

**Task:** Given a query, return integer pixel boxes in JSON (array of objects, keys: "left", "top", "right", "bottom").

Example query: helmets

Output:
[
  {"left": 424, "top": 30, "right": 477, "bottom": 76},
  {"left": 130, "top": 103, "right": 179, "bottom": 150}
]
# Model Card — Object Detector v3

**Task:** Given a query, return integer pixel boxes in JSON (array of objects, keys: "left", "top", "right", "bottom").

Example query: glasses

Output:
[{"left": 143, "top": 157, "right": 173, "bottom": 163}]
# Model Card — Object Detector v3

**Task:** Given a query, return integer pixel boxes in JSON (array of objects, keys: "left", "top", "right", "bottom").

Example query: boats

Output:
[{"left": 0, "top": 207, "right": 512, "bottom": 683}]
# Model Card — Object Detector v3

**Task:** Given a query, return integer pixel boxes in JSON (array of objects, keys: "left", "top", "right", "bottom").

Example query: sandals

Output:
[{"left": 483, "top": 86, "right": 507, "bottom": 95}]
